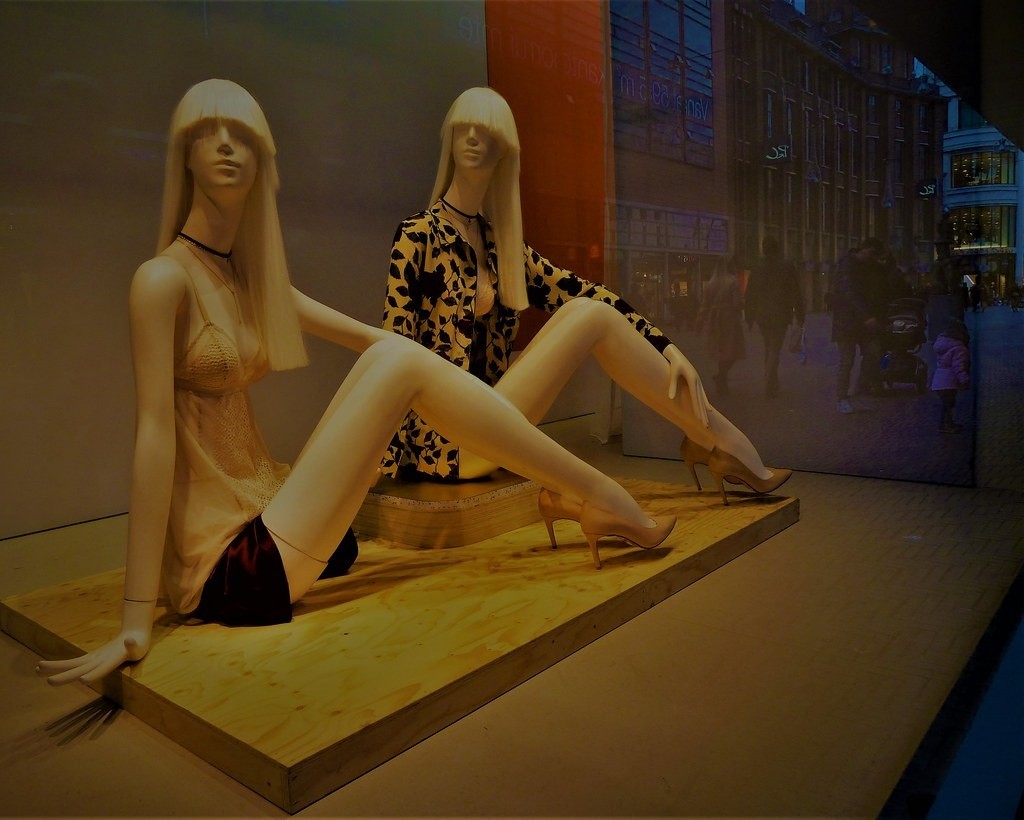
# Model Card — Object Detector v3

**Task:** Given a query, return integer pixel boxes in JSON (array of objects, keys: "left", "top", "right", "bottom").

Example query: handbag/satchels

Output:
[{"left": 788, "top": 323, "right": 804, "bottom": 354}]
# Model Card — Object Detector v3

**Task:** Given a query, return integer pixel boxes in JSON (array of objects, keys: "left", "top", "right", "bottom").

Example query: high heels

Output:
[
  {"left": 580, "top": 500, "right": 677, "bottom": 569},
  {"left": 709, "top": 446, "right": 794, "bottom": 504},
  {"left": 680, "top": 436, "right": 745, "bottom": 491},
  {"left": 538, "top": 485, "right": 582, "bottom": 550}
]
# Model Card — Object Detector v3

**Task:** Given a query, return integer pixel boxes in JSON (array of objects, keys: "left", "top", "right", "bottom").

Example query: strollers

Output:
[{"left": 872, "top": 296, "right": 929, "bottom": 394}]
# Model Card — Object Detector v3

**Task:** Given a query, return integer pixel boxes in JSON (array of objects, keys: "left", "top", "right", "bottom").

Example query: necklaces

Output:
[
  {"left": 441, "top": 197, "right": 480, "bottom": 253},
  {"left": 176, "top": 232, "right": 241, "bottom": 324}
]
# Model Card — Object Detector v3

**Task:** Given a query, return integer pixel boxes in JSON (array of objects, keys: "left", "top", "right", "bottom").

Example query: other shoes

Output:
[
  {"left": 713, "top": 373, "right": 729, "bottom": 396},
  {"left": 836, "top": 398, "right": 854, "bottom": 414},
  {"left": 938, "top": 422, "right": 965, "bottom": 435},
  {"left": 859, "top": 400, "right": 879, "bottom": 412}
]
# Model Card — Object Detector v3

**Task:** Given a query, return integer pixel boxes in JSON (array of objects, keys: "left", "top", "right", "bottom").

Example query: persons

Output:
[
  {"left": 693, "top": 233, "right": 1020, "bottom": 432},
  {"left": 35, "top": 79, "right": 678, "bottom": 686},
  {"left": 377, "top": 85, "right": 792, "bottom": 505}
]
